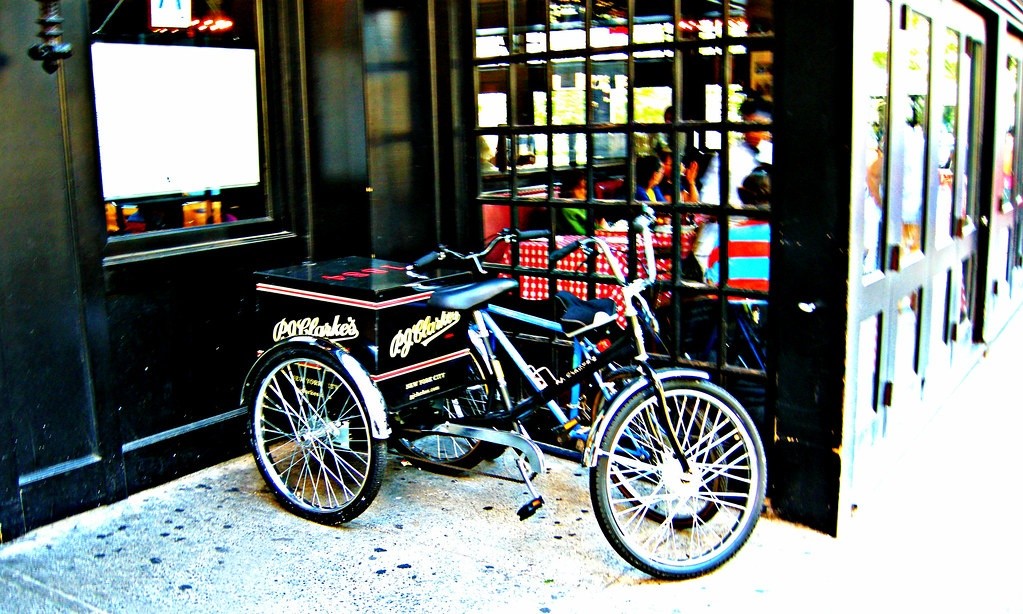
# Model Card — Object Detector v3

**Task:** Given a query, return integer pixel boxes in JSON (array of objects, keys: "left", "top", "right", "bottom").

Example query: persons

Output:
[
  {"left": 650, "top": 106, "right": 672, "bottom": 154},
  {"left": 700, "top": 97, "right": 772, "bottom": 203},
  {"left": 633, "top": 156, "right": 670, "bottom": 225},
  {"left": 558, "top": 170, "right": 609, "bottom": 234},
  {"left": 705, "top": 171, "right": 770, "bottom": 301},
  {"left": 660, "top": 152, "right": 700, "bottom": 203}
]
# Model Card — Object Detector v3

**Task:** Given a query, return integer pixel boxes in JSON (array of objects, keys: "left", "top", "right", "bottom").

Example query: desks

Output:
[{"left": 497, "top": 223, "right": 701, "bottom": 332}]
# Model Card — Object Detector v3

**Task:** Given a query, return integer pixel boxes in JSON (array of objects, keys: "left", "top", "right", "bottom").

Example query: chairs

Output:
[{"left": 563, "top": 207, "right": 601, "bottom": 235}]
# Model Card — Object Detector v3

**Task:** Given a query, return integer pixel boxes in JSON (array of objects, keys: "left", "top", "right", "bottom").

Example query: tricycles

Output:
[{"left": 232, "top": 208, "right": 768, "bottom": 582}]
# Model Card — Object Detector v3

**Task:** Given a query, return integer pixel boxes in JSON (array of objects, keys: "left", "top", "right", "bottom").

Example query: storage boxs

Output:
[{"left": 246, "top": 255, "right": 474, "bottom": 420}]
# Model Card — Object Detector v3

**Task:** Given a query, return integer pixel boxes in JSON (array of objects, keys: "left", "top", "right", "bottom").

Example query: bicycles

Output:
[{"left": 383, "top": 230, "right": 729, "bottom": 533}]
